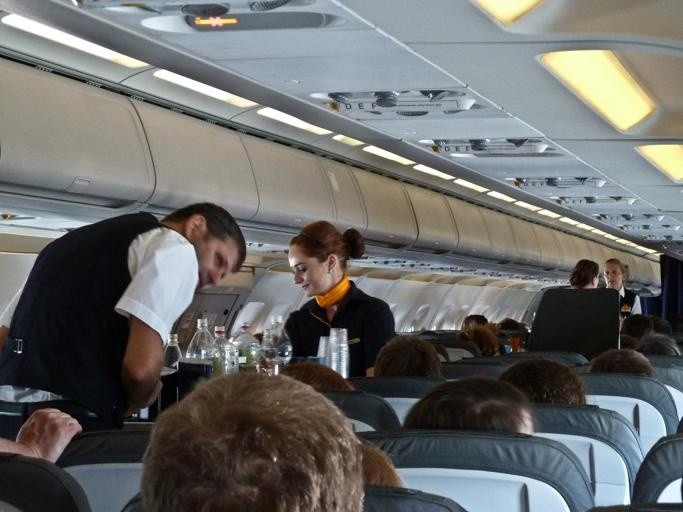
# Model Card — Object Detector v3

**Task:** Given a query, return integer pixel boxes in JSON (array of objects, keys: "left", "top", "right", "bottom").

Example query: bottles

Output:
[
  {"left": 230, "top": 321, "right": 262, "bottom": 375},
  {"left": 255, "top": 327, "right": 279, "bottom": 378},
  {"left": 185, "top": 319, "right": 222, "bottom": 381},
  {"left": 267, "top": 314, "right": 292, "bottom": 365},
  {"left": 212, "top": 324, "right": 239, "bottom": 376},
  {"left": 162, "top": 333, "right": 184, "bottom": 374}
]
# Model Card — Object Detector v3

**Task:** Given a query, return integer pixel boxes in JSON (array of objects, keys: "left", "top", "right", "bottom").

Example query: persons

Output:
[
  {"left": 498, "top": 357, "right": 586, "bottom": 406},
  {"left": 621, "top": 314, "right": 651, "bottom": 335},
  {"left": 139, "top": 371, "right": 365, "bottom": 511},
  {"left": 373, "top": 334, "right": 441, "bottom": 378},
  {"left": 570, "top": 259, "right": 600, "bottom": 289},
  {"left": 648, "top": 315, "right": 672, "bottom": 334},
  {"left": 620, "top": 335, "right": 640, "bottom": 348},
  {"left": 456, "top": 326, "right": 499, "bottom": 357},
  {"left": 0, "top": 407, "right": 85, "bottom": 464},
  {"left": 278, "top": 363, "right": 356, "bottom": 393},
  {"left": 401, "top": 377, "right": 534, "bottom": 435},
  {"left": 603, "top": 258, "right": 642, "bottom": 333},
  {"left": 281, "top": 219, "right": 395, "bottom": 378},
  {"left": 637, "top": 332, "right": 680, "bottom": 357},
  {"left": 589, "top": 349, "right": 653, "bottom": 376},
  {"left": 0, "top": 203, "right": 247, "bottom": 469}
]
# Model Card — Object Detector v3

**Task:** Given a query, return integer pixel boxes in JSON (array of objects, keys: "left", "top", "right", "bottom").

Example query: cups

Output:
[
  {"left": 326, "top": 327, "right": 350, "bottom": 380},
  {"left": 316, "top": 335, "right": 329, "bottom": 366}
]
